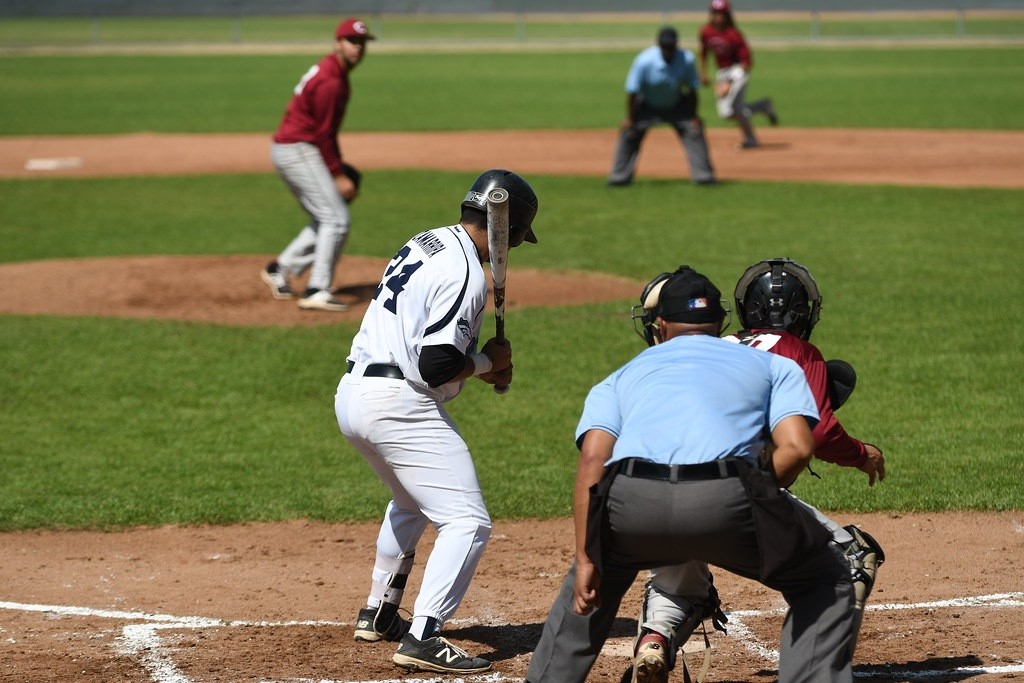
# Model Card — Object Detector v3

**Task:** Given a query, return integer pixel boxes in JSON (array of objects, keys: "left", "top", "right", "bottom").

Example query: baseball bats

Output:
[{"left": 485, "top": 186, "right": 511, "bottom": 394}]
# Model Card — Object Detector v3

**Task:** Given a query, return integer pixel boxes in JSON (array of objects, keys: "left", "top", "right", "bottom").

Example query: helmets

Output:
[
  {"left": 709, "top": 0, "right": 730, "bottom": 15},
  {"left": 735, "top": 257, "right": 822, "bottom": 340},
  {"left": 462, "top": 169, "right": 539, "bottom": 244}
]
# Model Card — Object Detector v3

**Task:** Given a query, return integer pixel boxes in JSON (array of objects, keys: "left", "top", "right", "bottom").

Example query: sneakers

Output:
[
  {"left": 634, "top": 632, "right": 669, "bottom": 683},
  {"left": 391, "top": 633, "right": 493, "bottom": 674},
  {"left": 352, "top": 607, "right": 412, "bottom": 641},
  {"left": 260, "top": 261, "right": 294, "bottom": 300},
  {"left": 297, "top": 288, "right": 348, "bottom": 311}
]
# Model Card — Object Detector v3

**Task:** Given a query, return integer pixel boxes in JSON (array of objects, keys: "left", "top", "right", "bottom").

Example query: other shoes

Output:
[
  {"left": 765, "top": 99, "right": 779, "bottom": 125},
  {"left": 738, "top": 138, "right": 758, "bottom": 149}
]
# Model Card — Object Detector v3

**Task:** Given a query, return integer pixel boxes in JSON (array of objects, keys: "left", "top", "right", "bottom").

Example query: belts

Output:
[
  {"left": 616, "top": 459, "right": 739, "bottom": 480},
  {"left": 347, "top": 361, "right": 404, "bottom": 380}
]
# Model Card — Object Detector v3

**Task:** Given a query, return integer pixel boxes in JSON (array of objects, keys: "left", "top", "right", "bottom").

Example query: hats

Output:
[
  {"left": 658, "top": 29, "right": 677, "bottom": 49},
  {"left": 335, "top": 20, "right": 374, "bottom": 41},
  {"left": 656, "top": 265, "right": 725, "bottom": 323}
]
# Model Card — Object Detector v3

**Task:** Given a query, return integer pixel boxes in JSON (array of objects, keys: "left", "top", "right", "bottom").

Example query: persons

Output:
[
  {"left": 261, "top": 19, "right": 376, "bottom": 312},
  {"left": 699, "top": 0, "right": 779, "bottom": 149},
  {"left": 602, "top": 25, "right": 714, "bottom": 186},
  {"left": 526, "top": 257, "right": 886, "bottom": 683},
  {"left": 334, "top": 170, "right": 538, "bottom": 674}
]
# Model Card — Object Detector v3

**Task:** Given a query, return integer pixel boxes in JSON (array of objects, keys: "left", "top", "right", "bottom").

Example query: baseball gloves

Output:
[
  {"left": 824, "top": 358, "right": 857, "bottom": 412},
  {"left": 340, "top": 161, "right": 363, "bottom": 203},
  {"left": 715, "top": 78, "right": 733, "bottom": 97}
]
